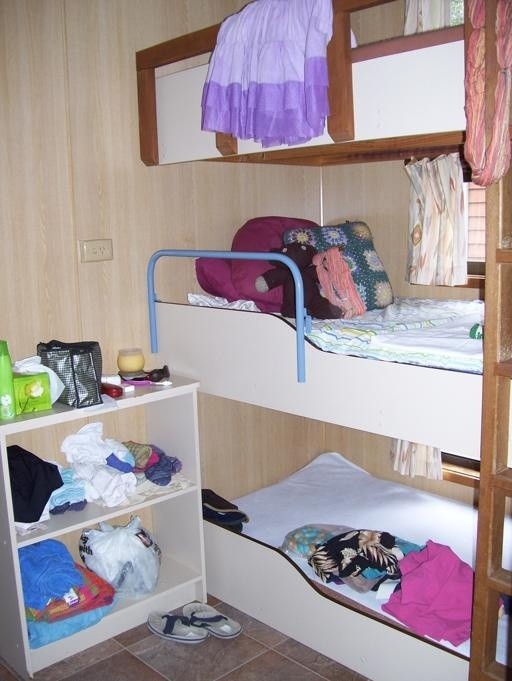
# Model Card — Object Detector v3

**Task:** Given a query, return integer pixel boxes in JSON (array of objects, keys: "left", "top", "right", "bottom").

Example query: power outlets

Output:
[{"left": 81, "top": 238, "right": 114, "bottom": 263}]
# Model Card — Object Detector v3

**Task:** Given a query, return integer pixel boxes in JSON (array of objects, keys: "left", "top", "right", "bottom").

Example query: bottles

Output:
[{"left": 0, "top": 339, "right": 16, "bottom": 424}]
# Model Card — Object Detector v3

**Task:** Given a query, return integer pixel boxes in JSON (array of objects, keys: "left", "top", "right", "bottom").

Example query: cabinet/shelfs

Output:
[{"left": 0, "top": 375, "right": 208, "bottom": 681}]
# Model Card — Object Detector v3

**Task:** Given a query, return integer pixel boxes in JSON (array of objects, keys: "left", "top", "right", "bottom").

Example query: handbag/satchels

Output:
[
  {"left": 201, "top": 489, "right": 249, "bottom": 534},
  {"left": 36, "top": 339, "right": 104, "bottom": 408}
]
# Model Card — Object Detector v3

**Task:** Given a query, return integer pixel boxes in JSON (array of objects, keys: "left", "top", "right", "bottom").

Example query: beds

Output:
[{"left": 135, "top": 0, "right": 512, "bottom": 681}]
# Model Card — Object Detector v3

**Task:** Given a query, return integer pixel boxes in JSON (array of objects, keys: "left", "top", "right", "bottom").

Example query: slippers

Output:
[{"left": 147, "top": 602, "right": 243, "bottom": 646}]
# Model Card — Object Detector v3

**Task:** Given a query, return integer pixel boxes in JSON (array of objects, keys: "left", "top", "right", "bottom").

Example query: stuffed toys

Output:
[{"left": 255, "top": 240, "right": 342, "bottom": 320}]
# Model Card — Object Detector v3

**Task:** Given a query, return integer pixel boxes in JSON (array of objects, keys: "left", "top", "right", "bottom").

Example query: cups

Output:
[{"left": 117, "top": 348, "right": 145, "bottom": 375}]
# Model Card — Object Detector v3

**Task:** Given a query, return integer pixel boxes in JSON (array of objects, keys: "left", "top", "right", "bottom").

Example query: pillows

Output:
[{"left": 194, "top": 214, "right": 394, "bottom": 319}]
